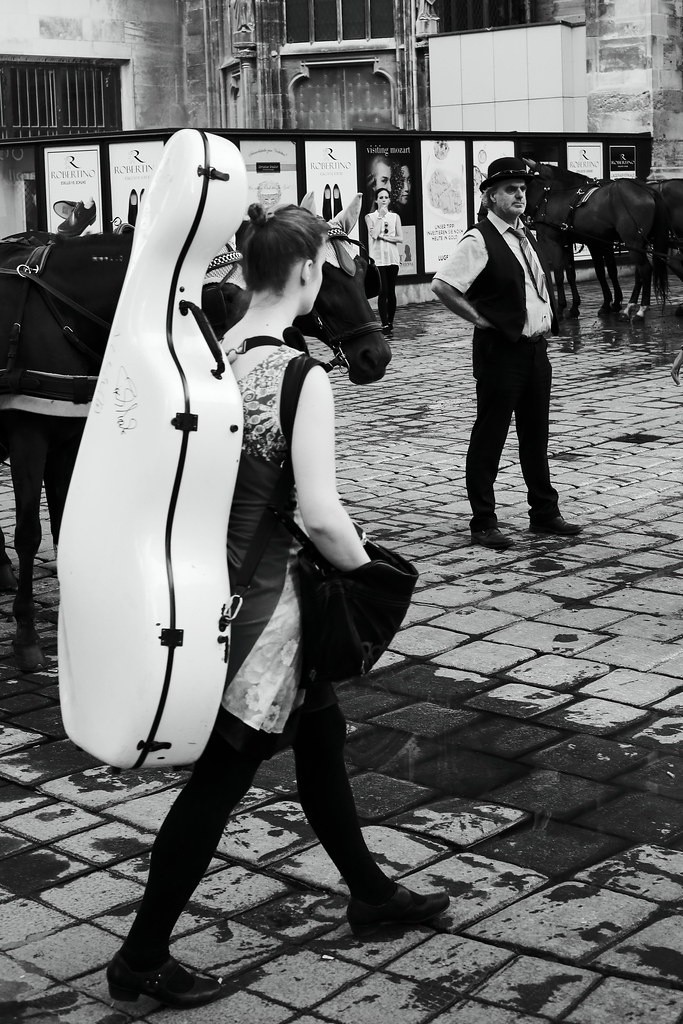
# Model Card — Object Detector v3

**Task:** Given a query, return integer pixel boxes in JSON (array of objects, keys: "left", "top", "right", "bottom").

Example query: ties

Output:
[{"left": 506, "top": 227, "right": 547, "bottom": 304}]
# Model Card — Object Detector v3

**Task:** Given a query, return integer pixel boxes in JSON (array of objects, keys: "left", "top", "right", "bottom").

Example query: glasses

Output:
[{"left": 383, "top": 221, "right": 388, "bottom": 233}]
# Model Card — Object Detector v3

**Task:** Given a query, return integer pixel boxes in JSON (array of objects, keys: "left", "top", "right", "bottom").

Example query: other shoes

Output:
[{"left": 382, "top": 325, "right": 394, "bottom": 337}]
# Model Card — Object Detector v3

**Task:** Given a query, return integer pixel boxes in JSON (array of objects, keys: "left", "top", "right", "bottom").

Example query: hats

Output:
[{"left": 479, "top": 157, "right": 535, "bottom": 195}]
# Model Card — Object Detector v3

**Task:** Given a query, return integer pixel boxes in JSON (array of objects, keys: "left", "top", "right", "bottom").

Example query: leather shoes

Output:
[
  {"left": 471, "top": 527, "right": 512, "bottom": 549},
  {"left": 529, "top": 515, "right": 583, "bottom": 535}
]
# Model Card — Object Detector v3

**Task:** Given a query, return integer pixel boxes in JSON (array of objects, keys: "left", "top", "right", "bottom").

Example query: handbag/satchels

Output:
[{"left": 273, "top": 352, "right": 418, "bottom": 692}]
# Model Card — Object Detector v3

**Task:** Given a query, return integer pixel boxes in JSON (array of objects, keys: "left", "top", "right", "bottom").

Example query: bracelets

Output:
[{"left": 377, "top": 217, "right": 383, "bottom": 219}]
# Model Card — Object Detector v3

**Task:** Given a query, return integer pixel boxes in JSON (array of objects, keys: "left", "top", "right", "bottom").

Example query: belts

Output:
[{"left": 517, "top": 335, "right": 542, "bottom": 346}]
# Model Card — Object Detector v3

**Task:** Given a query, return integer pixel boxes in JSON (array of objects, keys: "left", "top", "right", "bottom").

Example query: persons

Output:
[
  {"left": 397, "top": 165, "right": 411, "bottom": 204},
  {"left": 365, "top": 189, "right": 403, "bottom": 334},
  {"left": 367, "top": 158, "right": 395, "bottom": 204},
  {"left": 108, "top": 203, "right": 449, "bottom": 1006},
  {"left": 430, "top": 155, "right": 583, "bottom": 550}
]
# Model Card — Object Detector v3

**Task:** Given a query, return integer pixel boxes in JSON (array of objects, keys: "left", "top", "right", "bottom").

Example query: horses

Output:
[
  {"left": 1, "top": 192, "right": 393, "bottom": 673},
  {"left": 477, "top": 157, "right": 682, "bottom": 322}
]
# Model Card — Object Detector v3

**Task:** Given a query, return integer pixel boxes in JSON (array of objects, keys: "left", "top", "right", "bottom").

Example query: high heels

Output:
[
  {"left": 346, "top": 884, "right": 449, "bottom": 937},
  {"left": 106, "top": 952, "right": 229, "bottom": 1008}
]
